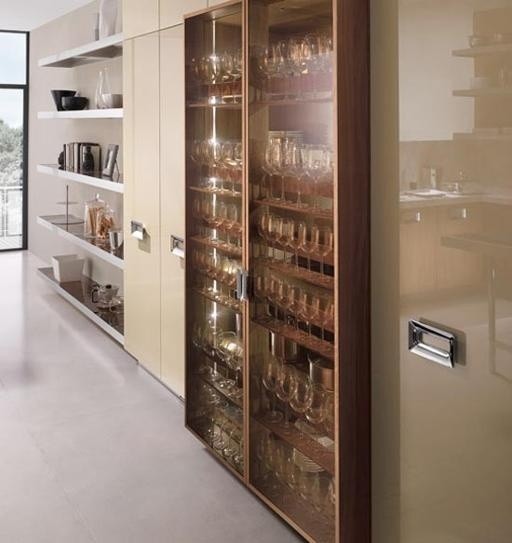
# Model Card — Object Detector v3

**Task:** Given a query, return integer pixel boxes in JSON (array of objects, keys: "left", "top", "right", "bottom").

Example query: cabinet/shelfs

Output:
[
  {"left": 401, "top": 204, "right": 480, "bottom": 305},
  {"left": 482, "top": 204, "right": 512, "bottom": 299},
  {"left": 36, "top": 33, "right": 124, "bottom": 343},
  {"left": 183, "top": 0, "right": 370, "bottom": 542}
]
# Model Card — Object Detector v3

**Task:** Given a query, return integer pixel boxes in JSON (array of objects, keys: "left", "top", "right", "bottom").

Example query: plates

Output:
[{"left": 268, "top": 130, "right": 303, "bottom": 148}]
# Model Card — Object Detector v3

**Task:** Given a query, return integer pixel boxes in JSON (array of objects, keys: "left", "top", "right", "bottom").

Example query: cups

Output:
[
  {"left": 258, "top": 436, "right": 336, "bottom": 543},
  {"left": 324, "top": 395, "right": 335, "bottom": 466},
  {"left": 214, "top": 401, "right": 227, "bottom": 451}
]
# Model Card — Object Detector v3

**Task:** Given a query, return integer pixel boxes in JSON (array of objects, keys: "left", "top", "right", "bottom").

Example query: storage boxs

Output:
[{"left": 51, "top": 254, "right": 84, "bottom": 283}]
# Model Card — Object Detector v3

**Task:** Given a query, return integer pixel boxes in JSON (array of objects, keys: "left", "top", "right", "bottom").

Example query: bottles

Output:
[
  {"left": 96, "top": 202, "right": 115, "bottom": 239},
  {"left": 95, "top": 68, "right": 112, "bottom": 109},
  {"left": 84, "top": 194, "right": 104, "bottom": 239}
]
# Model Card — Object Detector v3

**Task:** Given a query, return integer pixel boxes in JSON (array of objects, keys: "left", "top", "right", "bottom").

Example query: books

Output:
[{"left": 63, "top": 142, "right": 100, "bottom": 174}]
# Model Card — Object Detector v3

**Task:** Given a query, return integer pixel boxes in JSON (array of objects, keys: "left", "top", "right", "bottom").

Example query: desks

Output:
[{"left": 440, "top": 232, "right": 511, "bottom": 382}]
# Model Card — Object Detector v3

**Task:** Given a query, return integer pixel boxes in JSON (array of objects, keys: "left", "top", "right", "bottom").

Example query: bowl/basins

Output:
[
  {"left": 103, "top": 92, "right": 123, "bottom": 109},
  {"left": 62, "top": 97, "right": 88, "bottom": 110},
  {"left": 49, "top": 91, "right": 77, "bottom": 111}
]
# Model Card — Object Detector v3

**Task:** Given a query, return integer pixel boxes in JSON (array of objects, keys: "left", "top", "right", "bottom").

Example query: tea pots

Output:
[
  {"left": 92, "top": 284, "right": 118, "bottom": 307},
  {"left": 108, "top": 296, "right": 126, "bottom": 313}
]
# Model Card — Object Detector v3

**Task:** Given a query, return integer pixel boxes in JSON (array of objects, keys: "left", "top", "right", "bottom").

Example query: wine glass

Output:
[
  {"left": 266, "top": 278, "right": 282, "bottom": 329},
  {"left": 259, "top": 214, "right": 271, "bottom": 267},
  {"left": 312, "top": 227, "right": 332, "bottom": 284},
  {"left": 303, "top": 384, "right": 328, "bottom": 456},
  {"left": 299, "top": 292, "right": 316, "bottom": 343},
  {"left": 262, "top": 356, "right": 284, "bottom": 423},
  {"left": 304, "top": 145, "right": 327, "bottom": 216},
  {"left": 272, "top": 143, "right": 293, "bottom": 205},
  {"left": 261, "top": 141, "right": 277, "bottom": 202},
  {"left": 287, "top": 221, "right": 304, "bottom": 277},
  {"left": 300, "top": 224, "right": 318, "bottom": 280},
  {"left": 192, "top": 136, "right": 243, "bottom": 195},
  {"left": 292, "top": 142, "right": 310, "bottom": 209},
  {"left": 254, "top": 275, "right": 271, "bottom": 324},
  {"left": 191, "top": 49, "right": 242, "bottom": 104},
  {"left": 191, "top": 199, "right": 244, "bottom": 250},
  {"left": 276, "top": 366, "right": 297, "bottom": 438},
  {"left": 216, "top": 331, "right": 235, "bottom": 388},
  {"left": 226, "top": 336, "right": 243, "bottom": 399},
  {"left": 206, "top": 396, "right": 220, "bottom": 441},
  {"left": 193, "top": 322, "right": 210, "bottom": 376},
  {"left": 258, "top": 31, "right": 333, "bottom": 95},
  {"left": 290, "top": 376, "right": 313, "bottom": 450},
  {"left": 202, "top": 326, "right": 221, "bottom": 381},
  {"left": 233, "top": 419, "right": 244, "bottom": 465},
  {"left": 312, "top": 297, "right": 332, "bottom": 352},
  {"left": 193, "top": 250, "right": 242, "bottom": 312},
  {"left": 276, "top": 219, "right": 293, "bottom": 275},
  {"left": 268, "top": 216, "right": 283, "bottom": 268},
  {"left": 222, "top": 408, "right": 237, "bottom": 458},
  {"left": 287, "top": 285, "right": 304, "bottom": 339},
  {"left": 275, "top": 283, "right": 293, "bottom": 334}
]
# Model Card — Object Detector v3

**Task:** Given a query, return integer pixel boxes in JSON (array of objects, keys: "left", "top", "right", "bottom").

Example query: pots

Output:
[
  {"left": 269, "top": 329, "right": 310, "bottom": 365},
  {"left": 306, "top": 354, "right": 334, "bottom": 392}
]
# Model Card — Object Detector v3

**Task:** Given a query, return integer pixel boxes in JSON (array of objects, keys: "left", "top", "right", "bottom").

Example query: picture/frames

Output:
[{"left": 102, "top": 143, "right": 119, "bottom": 176}]
